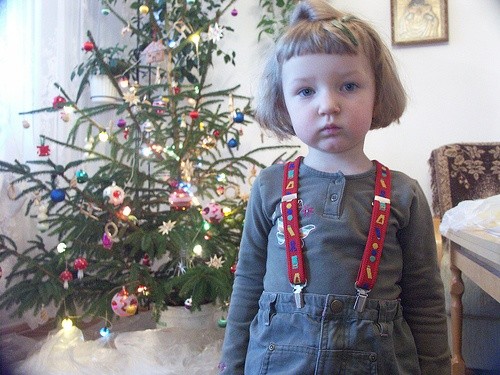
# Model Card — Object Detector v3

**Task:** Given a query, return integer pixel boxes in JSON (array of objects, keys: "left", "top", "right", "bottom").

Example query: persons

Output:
[{"left": 219, "top": 1, "right": 454, "bottom": 375}]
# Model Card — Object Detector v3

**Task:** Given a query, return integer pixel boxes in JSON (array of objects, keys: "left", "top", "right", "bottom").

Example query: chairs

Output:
[{"left": 427, "top": 141, "right": 500, "bottom": 272}]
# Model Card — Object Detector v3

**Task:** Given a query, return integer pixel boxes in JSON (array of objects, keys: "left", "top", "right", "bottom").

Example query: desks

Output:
[{"left": 443, "top": 229, "right": 500, "bottom": 375}]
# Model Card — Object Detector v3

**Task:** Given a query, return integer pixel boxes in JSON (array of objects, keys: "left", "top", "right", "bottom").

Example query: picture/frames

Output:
[{"left": 390, "top": 0, "right": 448, "bottom": 48}]
